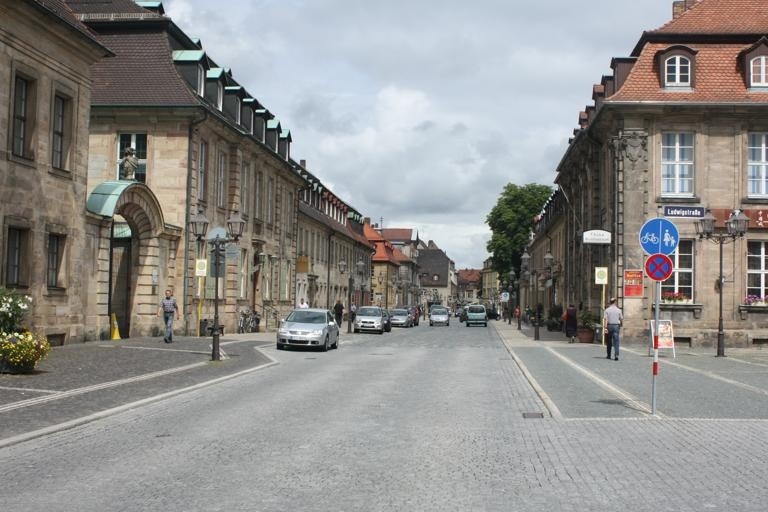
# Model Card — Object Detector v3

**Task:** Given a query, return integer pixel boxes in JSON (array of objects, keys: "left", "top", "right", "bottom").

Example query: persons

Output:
[
  {"left": 602, "top": 297, "right": 624, "bottom": 361},
  {"left": 155, "top": 289, "right": 181, "bottom": 345},
  {"left": 412, "top": 300, "right": 532, "bottom": 327},
  {"left": 333, "top": 299, "right": 345, "bottom": 328},
  {"left": 248, "top": 319, "right": 260, "bottom": 332},
  {"left": 299, "top": 298, "right": 310, "bottom": 309},
  {"left": 350, "top": 302, "right": 357, "bottom": 323},
  {"left": 560, "top": 303, "right": 579, "bottom": 343},
  {"left": 248, "top": 310, "right": 261, "bottom": 333}
]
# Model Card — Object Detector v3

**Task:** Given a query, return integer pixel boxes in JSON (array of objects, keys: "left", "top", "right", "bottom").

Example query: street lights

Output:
[
  {"left": 337, "top": 256, "right": 365, "bottom": 334},
  {"left": 188, "top": 204, "right": 246, "bottom": 363},
  {"left": 521, "top": 245, "right": 557, "bottom": 341},
  {"left": 508, "top": 266, "right": 530, "bottom": 331},
  {"left": 377, "top": 270, "right": 399, "bottom": 310},
  {"left": 502, "top": 280, "right": 519, "bottom": 324},
  {"left": 258, "top": 251, "right": 280, "bottom": 328},
  {"left": 692, "top": 207, "right": 751, "bottom": 359}
]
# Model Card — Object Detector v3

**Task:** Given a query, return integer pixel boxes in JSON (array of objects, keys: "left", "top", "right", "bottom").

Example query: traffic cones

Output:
[{"left": 109, "top": 312, "right": 122, "bottom": 340}]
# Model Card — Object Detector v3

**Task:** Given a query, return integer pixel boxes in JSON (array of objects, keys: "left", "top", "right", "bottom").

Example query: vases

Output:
[{"left": 1, "top": 360, "right": 29, "bottom": 373}]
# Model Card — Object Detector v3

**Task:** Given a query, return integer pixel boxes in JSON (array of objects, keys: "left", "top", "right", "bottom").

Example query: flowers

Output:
[
  {"left": 0, "top": 329, "right": 52, "bottom": 369},
  {"left": 661, "top": 292, "right": 687, "bottom": 302},
  {"left": 744, "top": 295, "right": 768, "bottom": 305}
]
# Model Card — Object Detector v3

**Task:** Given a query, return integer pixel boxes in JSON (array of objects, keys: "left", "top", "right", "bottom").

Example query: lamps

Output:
[
  {"left": 270, "top": 256, "right": 277, "bottom": 263},
  {"left": 259, "top": 252, "right": 265, "bottom": 263}
]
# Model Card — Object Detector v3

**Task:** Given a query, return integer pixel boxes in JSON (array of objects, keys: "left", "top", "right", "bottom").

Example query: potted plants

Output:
[{"left": 576, "top": 307, "right": 595, "bottom": 343}]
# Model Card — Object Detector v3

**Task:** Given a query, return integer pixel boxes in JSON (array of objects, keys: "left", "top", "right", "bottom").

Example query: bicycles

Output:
[{"left": 238, "top": 307, "right": 261, "bottom": 335}]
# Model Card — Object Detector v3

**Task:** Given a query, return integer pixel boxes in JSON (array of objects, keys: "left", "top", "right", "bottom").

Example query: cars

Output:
[
  {"left": 276, "top": 307, "right": 340, "bottom": 352},
  {"left": 426, "top": 304, "right": 501, "bottom": 328},
  {"left": 352, "top": 304, "right": 420, "bottom": 333}
]
